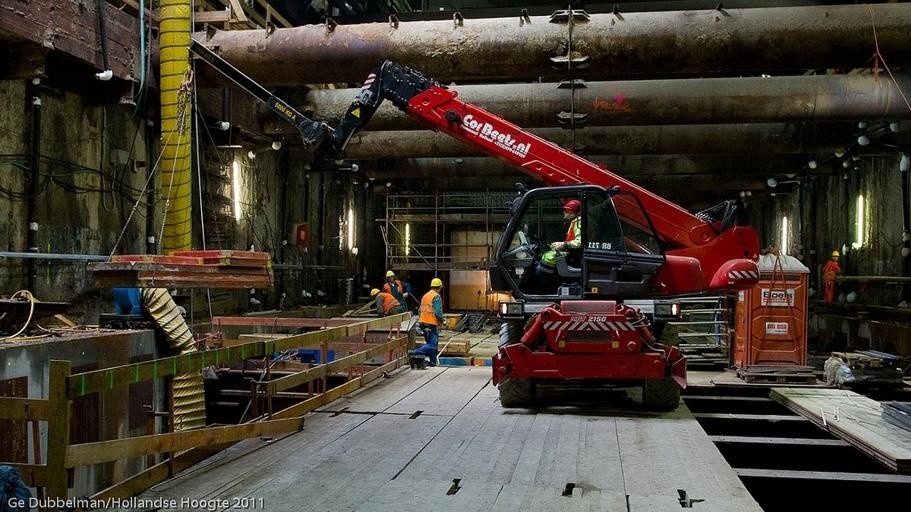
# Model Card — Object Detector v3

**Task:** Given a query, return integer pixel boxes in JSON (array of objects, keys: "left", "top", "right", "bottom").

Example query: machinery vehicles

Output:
[{"left": 188, "top": 35, "right": 760, "bottom": 414}]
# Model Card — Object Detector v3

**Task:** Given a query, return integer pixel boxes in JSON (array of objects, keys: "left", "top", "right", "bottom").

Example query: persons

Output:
[
  {"left": 370, "top": 287, "right": 402, "bottom": 317},
  {"left": 526, "top": 199, "right": 583, "bottom": 290},
  {"left": 383, "top": 270, "right": 411, "bottom": 314},
  {"left": 823, "top": 251, "right": 843, "bottom": 304},
  {"left": 407, "top": 278, "right": 448, "bottom": 366}
]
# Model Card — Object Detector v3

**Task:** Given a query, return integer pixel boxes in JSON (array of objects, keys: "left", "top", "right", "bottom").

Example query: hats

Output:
[
  {"left": 385, "top": 270, "right": 395, "bottom": 278},
  {"left": 562, "top": 198, "right": 580, "bottom": 212},
  {"left": 371, "top": 288, "right": 380, "bottom": 296},
  {"left": 429, "top": 277, "right": 441, "bottom": 287}
]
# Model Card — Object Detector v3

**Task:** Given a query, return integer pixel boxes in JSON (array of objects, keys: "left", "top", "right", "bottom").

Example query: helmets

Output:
[{"left": 831, "top": 251, "right": 839, "bottom": 256}]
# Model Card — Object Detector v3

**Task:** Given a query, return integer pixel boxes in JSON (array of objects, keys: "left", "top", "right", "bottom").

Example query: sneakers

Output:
[{"left": 407, "top": 349, "right": 416, "bottom": 366}]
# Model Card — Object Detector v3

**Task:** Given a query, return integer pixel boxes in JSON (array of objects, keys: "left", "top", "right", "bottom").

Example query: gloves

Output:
[{"left": 549, "top": 241, "right": 567, "bottom": 253}]
